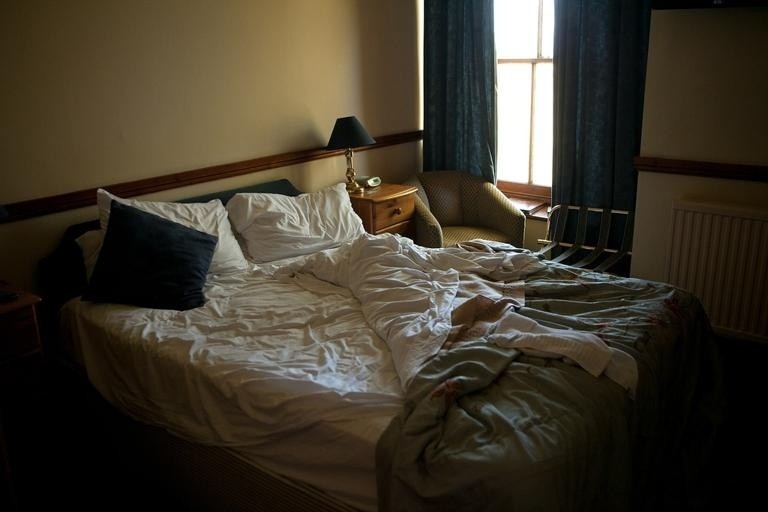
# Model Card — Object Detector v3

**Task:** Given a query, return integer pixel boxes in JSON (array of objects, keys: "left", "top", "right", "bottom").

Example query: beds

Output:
[{"left": 37, "top": 177, "right": 730, "bottom": 512}]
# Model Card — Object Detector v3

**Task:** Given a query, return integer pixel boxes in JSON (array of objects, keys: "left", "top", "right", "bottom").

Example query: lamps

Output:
[{"left": 326, "top": 115, "right": 376, "bottom": 194}]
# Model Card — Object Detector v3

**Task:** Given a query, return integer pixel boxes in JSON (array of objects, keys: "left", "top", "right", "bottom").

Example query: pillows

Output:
[{"left": 71, "top": 181, "right": 369, "bottom": 312}]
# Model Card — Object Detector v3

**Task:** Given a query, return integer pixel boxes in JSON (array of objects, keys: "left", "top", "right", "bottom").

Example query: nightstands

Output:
[
  {"left": 329, "top": 178, "right": 420, "bottom": 245},
  {"left": 0, "top": 283, "right": 46, "bottom": 381}
]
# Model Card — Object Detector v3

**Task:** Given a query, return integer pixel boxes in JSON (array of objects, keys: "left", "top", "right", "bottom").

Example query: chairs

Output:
[{"left": 401, "top": 169, "right": 527, "bottom": 249}]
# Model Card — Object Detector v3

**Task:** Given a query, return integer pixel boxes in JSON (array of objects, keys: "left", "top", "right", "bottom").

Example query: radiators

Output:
[{"left": 664, "top": 200, "right": 768, "bottom": 345}]
